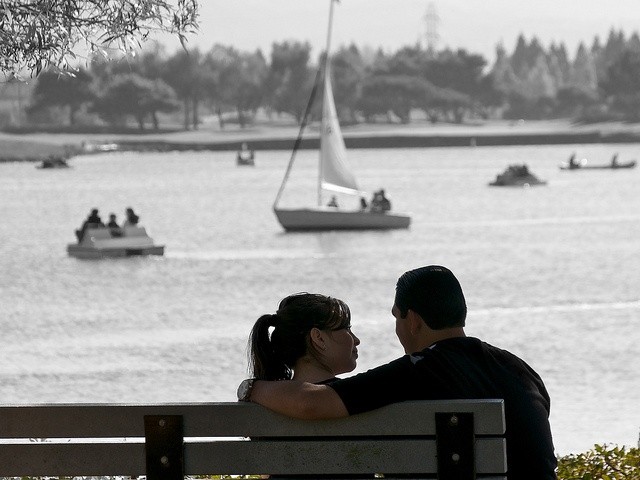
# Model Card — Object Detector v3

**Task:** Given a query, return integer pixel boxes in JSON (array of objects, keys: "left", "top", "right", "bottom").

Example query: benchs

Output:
[{"left": 1, "top": 397, "right": 509, "bottom": 480}]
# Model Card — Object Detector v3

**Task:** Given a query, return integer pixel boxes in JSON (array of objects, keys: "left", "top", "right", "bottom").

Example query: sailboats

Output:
[{"left": 273, "top": 0, "right": 412, "bottom": 230}]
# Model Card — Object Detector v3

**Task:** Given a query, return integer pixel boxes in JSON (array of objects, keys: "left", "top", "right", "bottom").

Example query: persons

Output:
[
  {"left": 247, "top": 291, "right": 376, "bottom": 479},
  {"left": 75, "top": 208, "right": 138, "bottom": 244},
  {"left": 239, "top": 265, "right": 557, "bottom": 479}
]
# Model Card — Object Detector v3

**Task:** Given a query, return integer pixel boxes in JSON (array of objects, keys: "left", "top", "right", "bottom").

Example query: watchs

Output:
[{"left": 237, "top": 377, "right": 259, "bottom": 401}]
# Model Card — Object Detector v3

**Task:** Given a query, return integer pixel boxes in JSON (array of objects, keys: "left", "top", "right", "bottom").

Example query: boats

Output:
[
  {"left": 68, "top": 226, "right": 164, "bottom": 259},
  {"left": 555, "top": 161, "right": 636, "bottom": 170}
]
